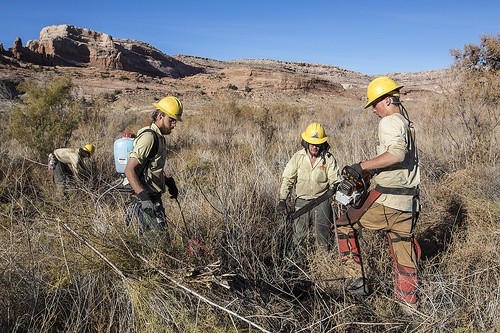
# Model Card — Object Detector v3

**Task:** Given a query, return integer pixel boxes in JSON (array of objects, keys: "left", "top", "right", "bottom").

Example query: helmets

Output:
[
  {"left": 363, "top": 77, "right": 404, "bottom": 110},
  {"left": 83, "top": 144, "right": 95, "bottom": 155},
  {"left": 301, "top": 122, "right": 330, "bottom": 145},
  {"left": 152, "top": 96, "right": 184, "bottom": 123}
]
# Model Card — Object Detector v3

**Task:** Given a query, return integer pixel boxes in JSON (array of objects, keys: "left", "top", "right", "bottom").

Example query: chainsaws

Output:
[{"left": 292, "top": 163, "right": 371, "bottom": 219}]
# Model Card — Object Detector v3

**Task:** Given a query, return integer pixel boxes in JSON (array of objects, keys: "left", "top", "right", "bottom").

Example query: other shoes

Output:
[{"left": 345, "top": 278, "right": 370, "bottom": 296}]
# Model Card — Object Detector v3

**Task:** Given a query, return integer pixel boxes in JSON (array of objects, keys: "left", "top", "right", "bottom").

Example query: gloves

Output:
[
  {"left": 164, "top": 176, "right": 178, "bottom": 199},
  {"left": 137, "top": 188, "right": 158, "bottom": 218},
  {"left": 276, "top": 199, "right": 287, "bottom": 214}
]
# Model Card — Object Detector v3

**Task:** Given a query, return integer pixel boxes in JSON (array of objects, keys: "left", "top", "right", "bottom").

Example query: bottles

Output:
[{"left": 49, "top": 155, "right": 55, "bottom": 166}]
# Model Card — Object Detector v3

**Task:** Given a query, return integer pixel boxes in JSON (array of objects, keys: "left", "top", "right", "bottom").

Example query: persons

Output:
[
  {"left": 122, "top": 96, "right": 184, "bottom": 277},
  {"left": 330, "top": 77, "right": 423, "bottom": 317},
  {"left": 274, "top": 123, "right": 342, "bottom": 264},
  {"left": 48, "top": 144, "right": 95, "bottom": 194}
]
